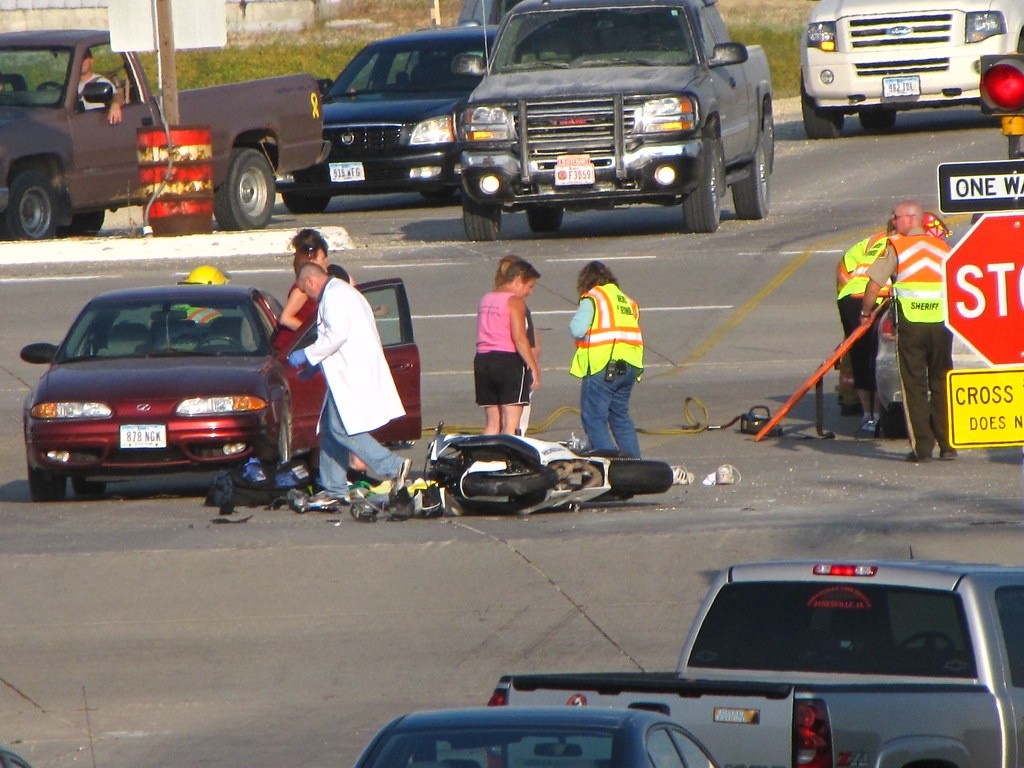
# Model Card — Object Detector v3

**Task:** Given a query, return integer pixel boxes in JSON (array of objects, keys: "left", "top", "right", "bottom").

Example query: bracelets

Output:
[{"left": 861, "top": 310, "right": 871, "bottom": 318}]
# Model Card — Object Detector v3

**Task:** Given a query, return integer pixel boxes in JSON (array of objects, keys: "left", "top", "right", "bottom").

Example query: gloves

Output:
[
  {"left": 287, "top": 349, "right": 307, "bottom": 369},
  {"left": 297, "top": 364, "right": 320, "bottom": 381}
]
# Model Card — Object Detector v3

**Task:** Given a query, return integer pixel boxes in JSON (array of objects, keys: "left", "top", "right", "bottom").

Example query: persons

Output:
[
  {"left": 860, "top": 201, "right": 961, "bottom": 462},
  {"left": 151, "top": 266, "right": 230, "bottom": 355},
  {"left": 473, "top": 254, "right": 540, "bottom": 438},
  {"left": 569, "top": 261, "right": 643, "bottom": 460},
  {"left": 78, "top": 47, "right": 123, "bottom": 126},
  {"left": 836, "top": 211, "right": 953, "bottom": 432},
  {"left": 273, "top": 228, "right": 413, "bottom": 509}
]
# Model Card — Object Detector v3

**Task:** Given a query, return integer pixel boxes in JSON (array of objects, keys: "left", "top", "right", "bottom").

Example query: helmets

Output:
[{"left": 177, "top": 265, "right": 231, "bottom": 286}]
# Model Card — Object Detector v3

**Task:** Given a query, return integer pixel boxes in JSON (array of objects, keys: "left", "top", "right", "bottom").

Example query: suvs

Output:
[
  {"left": 280, "top": 21, "right": 504, "bottom": 213},
  {"left": 800, "top": 0, "right": 1024, "bottom": 139}
]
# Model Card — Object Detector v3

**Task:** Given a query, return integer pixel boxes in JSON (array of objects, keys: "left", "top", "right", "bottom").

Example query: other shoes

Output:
[
  {"left": 906, "top": 452, "right": 933, "bottom": 463},
  {"left": 940, "top": 448, "right": 958, "bottom": 460},
  {"left": 839, "top": 401, "right": 865, "bottom": 416}
]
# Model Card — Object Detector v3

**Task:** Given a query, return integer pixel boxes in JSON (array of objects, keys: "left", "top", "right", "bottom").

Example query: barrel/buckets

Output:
[{"left": 135, "top": 124, "right": 213, "bottom": 237}]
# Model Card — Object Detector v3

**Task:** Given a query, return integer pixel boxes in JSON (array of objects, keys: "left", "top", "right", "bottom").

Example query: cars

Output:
[
  {"left": 356, "top": 706, "right": 719, "bottom": 768},
  {"left": 20, "top": 277, "right": 421, "bottom": 503}
]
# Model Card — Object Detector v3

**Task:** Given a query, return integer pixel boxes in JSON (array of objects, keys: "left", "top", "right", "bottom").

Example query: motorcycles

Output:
[{"left": 425, "top": 422, "right": 672, "bottom": 509}]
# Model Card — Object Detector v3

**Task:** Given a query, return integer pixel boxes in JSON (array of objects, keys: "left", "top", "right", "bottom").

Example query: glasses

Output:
[
  {"left": 306, "top": 244, "right": 314, "bottom": 261},
  {"left": 894, "top": 213, "right": 917, "bottom": 220}
]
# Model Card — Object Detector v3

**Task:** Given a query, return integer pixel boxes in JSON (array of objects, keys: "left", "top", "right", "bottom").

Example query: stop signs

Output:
[{"left": 942, "top": 211, "right": 1024, "bottom": 368}]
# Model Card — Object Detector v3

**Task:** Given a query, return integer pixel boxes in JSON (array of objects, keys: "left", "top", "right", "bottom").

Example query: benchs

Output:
[{"left": 103, "top": 320, "right": 208, "bottom": 350}]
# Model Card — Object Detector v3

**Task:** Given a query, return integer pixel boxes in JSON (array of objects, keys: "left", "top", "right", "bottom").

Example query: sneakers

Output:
[
  {"left": 305, "top": 488, "right": 353, "bottom": 508},
  {"left": 387, "top": 458, "right": 413, "bottom": 504}
]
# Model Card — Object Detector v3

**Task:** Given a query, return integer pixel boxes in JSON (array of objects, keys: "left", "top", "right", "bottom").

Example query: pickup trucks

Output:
[
  {"left": 449, "top": 0, "right": 773, "bottom": 241},
  {"left": 489, "top": 555, "right": 1024, "bottom": 768},
  {"left": 0, "top": 29, "right": 331, "bottom": 240}
]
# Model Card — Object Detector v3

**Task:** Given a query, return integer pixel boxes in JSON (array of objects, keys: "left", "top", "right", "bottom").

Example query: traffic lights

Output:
[{"left": 979, "top": 55, "right": 1024, "bottom": 116}]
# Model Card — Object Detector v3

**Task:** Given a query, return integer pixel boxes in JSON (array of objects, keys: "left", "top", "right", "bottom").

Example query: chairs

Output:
[
  {"left": 536, "top": 40, "right": 575, "bottom": 63},
  {"left": 205, "top": 314, "right": 243, "bottom": 347},
  {"left": 0, "top": 73, "right": 28, "bottom": 91},
  {"left": 98, "top": 322, "right": 147, "bottom": 357}
]
